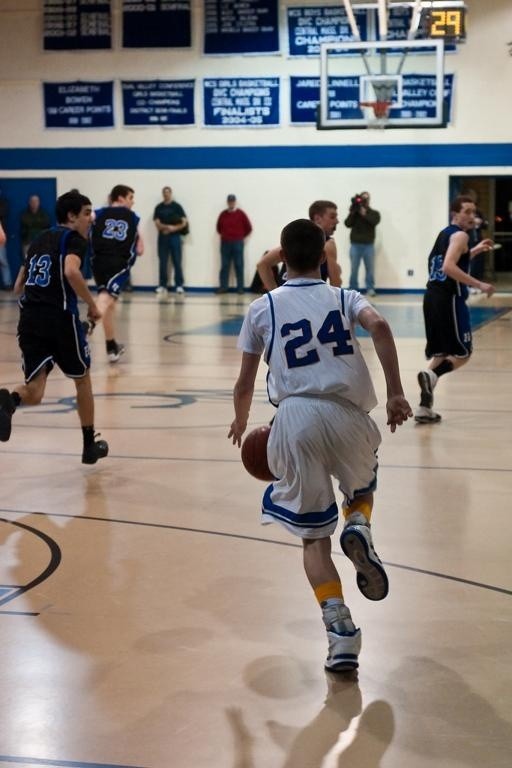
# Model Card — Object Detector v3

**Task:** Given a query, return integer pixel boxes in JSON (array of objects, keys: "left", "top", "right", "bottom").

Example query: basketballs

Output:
[{"left": 241, "top": 425, "right": 278, "bottom": 481}]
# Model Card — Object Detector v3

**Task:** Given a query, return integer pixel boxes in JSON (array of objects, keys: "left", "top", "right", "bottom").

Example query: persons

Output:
[
  {"left": 82, "top": 185, "right": 140, "bottom": 362},
  {"left": 216, "top": 196, "right": 251, "bottom": 293},
  {"left": 256, "top": 201, "right": 342, "bottom": 291},
  {"left": 466, "top": 211, "right": 489, "bottom": 294},
  {"left": 153, "top": 188, "right": 188, "bottom": 293},
  {"left": 345, "top": 192, "right": 380, "bottom": 297},
  {"left": 416, "top": 198, "right": 496, "bottom": 423},
  {"left": 0, "top": 193, "right": 108, "bottom": 463},
  {"left": 227, "top": 219, "right": 413, "bottom": 671},
  {"left": 1, "top": 196, "right": 143, "bottom": 292}
]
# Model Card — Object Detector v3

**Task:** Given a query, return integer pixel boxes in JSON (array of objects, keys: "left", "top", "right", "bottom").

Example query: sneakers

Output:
[
  {"left": 105, "top": 340, "right": 125, "bottom": 362},
  {"left": 418, "top": 371, "right": 434, "bottom": 403},
  {"left": 323, "top": 626, "right": 361, "bottom": 671},
  {"left": 1, "top": 388, "right": 17, "bottom": 441},
  {"left": 340, "top": 523, "right": 389, "bottom": 602},
  {"left": 415, "top": 409, "right": 441, "bottom": 424},
  {"left": 82, "top": 440, "right": 109, "bottom": 464},
  {"left": 123, "top": 284, "right": 376, "bottom": 296}
]
